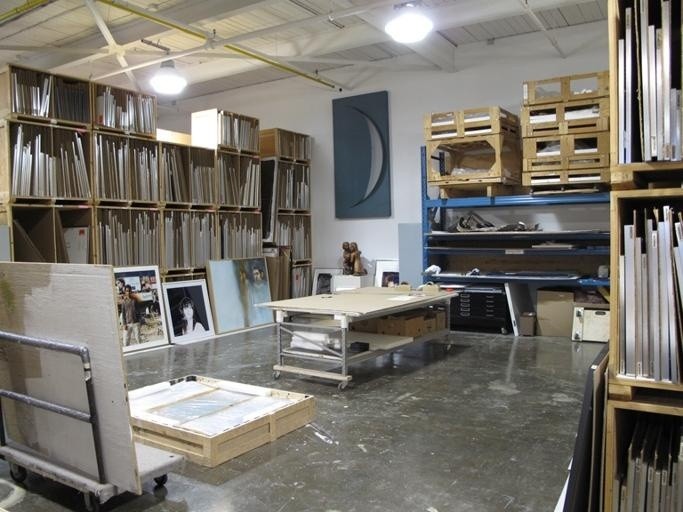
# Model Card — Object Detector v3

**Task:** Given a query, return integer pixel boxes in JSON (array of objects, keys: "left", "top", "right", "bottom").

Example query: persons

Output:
[
  {"left": 246, "top": 263, "right": 274, "bottom": 327},
  {"left": 176, "top": 297, "right": 206, "bottom": 336},
  {"left": 342, "top": 240, "right": 353, "bottom": 273},
  {"left": 119, "top": 284, "right": 141, "bottom": 347},
  {"left": 348, "top": 242, "right": 363, "bottom": 273}
]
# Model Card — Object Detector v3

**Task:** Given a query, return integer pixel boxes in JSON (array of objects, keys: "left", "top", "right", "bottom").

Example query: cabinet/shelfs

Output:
[{"left": 422, "top": 146, "right": 609, "bottom": 343}]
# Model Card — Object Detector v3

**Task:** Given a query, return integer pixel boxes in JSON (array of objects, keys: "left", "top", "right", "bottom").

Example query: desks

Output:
[{"left": 254, "top": 286, "right": 460, "bottom": 391}]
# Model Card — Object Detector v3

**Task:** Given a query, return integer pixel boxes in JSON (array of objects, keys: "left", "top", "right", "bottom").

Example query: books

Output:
[
  {"left": 12, "top": 68, "right": 312, "bottom": 297},
  {"left": 608, "top": 10, "right": 682, "bottom": 512}
]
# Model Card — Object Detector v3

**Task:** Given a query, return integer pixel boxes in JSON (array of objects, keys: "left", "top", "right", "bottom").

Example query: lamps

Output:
[
  {"left": 384, "top": 1, "right": 435, "bottom": 44},
  {"left": 142, "top": 40, "right": 186, "bottom": 95}
]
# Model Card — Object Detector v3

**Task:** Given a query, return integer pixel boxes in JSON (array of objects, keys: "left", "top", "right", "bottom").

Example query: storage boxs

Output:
[
  {"left": 536, "top": 288, "right": 574, "bottom": 337},
  {"left": 352, "top": 307, "right": 448, "bottom": 338}
]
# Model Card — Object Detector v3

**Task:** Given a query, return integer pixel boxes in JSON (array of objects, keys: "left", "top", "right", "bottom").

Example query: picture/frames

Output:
[
  {"left": 371, "top": 259, "right": 401, "bottom": 290},
  {"left": 313, "top": 268, "right": 342, "bottom": 298},
  {"left": 206, "top": 256, "right": 275, "bottom": 335},
  {"left": 161, "top": 279, "right": 218, "bottom": 344},
  {"left": 112, "top": 267, "right": 170, "bottom": 355}
]
